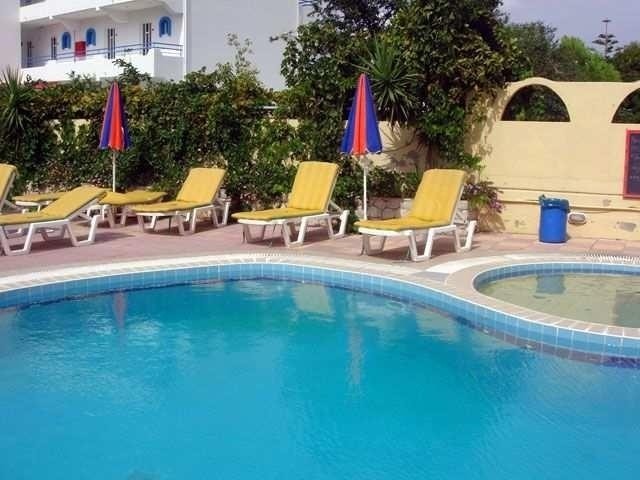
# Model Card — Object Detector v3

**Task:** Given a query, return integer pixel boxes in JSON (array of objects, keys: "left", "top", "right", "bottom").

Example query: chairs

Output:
[
  {"left": 230, "top": 160, "right": 349, "bottom": 248},
  {"left": 352, "top": 168, "right": 478, "bottom": 262},
  {"left": 0, "top": 163, "right": 231, "bottom": 256}
]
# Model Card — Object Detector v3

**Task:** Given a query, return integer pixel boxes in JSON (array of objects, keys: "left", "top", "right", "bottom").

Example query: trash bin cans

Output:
[{"left": 539, "top": 197, "right": 569, "bottom": 244}]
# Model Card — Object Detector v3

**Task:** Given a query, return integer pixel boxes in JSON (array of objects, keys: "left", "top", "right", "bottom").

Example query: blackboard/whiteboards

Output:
[{"left": 624, "top": 130, "right": 639, "bottom": 198}]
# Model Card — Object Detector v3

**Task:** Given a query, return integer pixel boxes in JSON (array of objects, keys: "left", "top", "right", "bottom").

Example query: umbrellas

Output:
[
  {"left": 96, "top": 76, "right": 131, "bottom": 196},
  {"left": 339, "top": 72, "right": 386, "bottom": 220}
]
